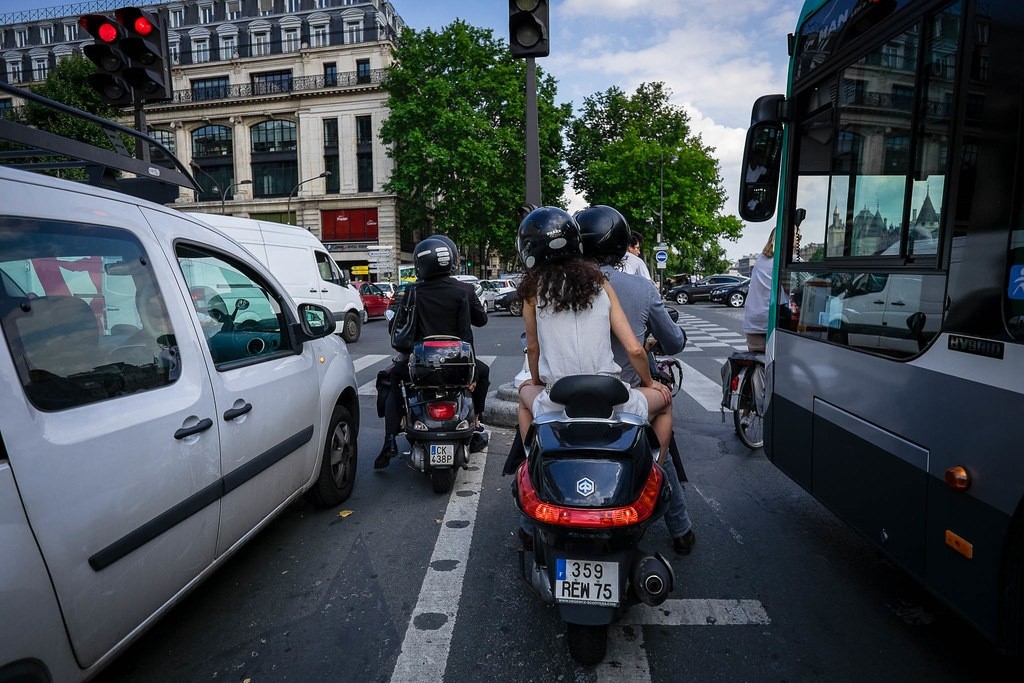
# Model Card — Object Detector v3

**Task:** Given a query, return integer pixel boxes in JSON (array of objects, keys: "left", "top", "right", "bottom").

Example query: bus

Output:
[{"left": 739, "top": 0, "right": 1024, "bottom": 668}]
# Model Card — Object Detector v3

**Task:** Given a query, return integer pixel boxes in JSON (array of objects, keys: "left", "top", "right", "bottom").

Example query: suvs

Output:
[{"left": 0, "top": 81, "right": 361, "bottom": 683}]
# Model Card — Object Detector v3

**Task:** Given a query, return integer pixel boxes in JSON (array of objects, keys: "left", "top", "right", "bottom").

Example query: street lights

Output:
[
  {"left": 288, "top": 171, "right": 332, "bottom": 226},
  {"left": 188, "top": 161, "right": 252, "bottom": 215},
  {"left": 646, "top": 152, "right": 679, "bottom": 299}
]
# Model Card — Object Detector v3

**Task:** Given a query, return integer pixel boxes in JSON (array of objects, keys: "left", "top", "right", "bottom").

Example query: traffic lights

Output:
[
  {"left": 79, "top": 12, "right": 133, "bottom": 107},
  {"left": 114, "top": 7, "right": 174, "bottom": 104},
  {"left": 509, "top": 0, "right": 550, "bottom": 57},
  {"left": 468, "top": 262, "right": 471, "bottom": 267}
]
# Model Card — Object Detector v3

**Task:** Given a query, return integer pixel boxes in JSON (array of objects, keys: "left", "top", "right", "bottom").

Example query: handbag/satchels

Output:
[
  {"left": 376, "top": 356, "right": 403, "bottom": 418},
  {"left": 391, "top": 283, "right": 416, "bottom": 353}
]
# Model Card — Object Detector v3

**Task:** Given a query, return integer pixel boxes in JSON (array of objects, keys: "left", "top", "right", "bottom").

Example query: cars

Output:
[
  {"left": 365, "top": 282, "right": 397, "bottom": 297},
  {"left": 388, "top": 284, "right": 409, "bottom": 311},
  {"left": 350, "top": 281, "right": 391, "bottom": 324},
  {"left": 665, "top": 274, "right": 751, "bottom": 308},
  {"left": 790, "top": 273, "right": 851, "bottom": 331},
  {"left": 450, "top": 273, "right": 523, "bottom": 317}
]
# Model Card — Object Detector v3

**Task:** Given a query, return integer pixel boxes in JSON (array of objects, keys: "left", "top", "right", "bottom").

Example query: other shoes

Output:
[
  {"left": 475, "top": 424, "right": 484, "bottom": 432},
  {"left": 674, "top": 528, "right": 695, "bottom": 555}
]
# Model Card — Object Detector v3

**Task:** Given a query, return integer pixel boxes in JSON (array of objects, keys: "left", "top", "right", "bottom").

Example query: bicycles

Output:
[{"left": 727, "top": 351, "right": 764, "bottom": 450}]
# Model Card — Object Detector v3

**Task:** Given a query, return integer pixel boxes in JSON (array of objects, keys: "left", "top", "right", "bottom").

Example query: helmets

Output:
[
  {"left": 575, "top": 204, "right": 631, "bottom": 259},
  {"left": 518, "top": 206, "right": 582, "bottom": 271},
  {"left": 413, "top": 234, "right": 458, "bottom": 279}
]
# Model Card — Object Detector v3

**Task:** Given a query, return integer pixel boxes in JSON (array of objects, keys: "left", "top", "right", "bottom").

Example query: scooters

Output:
[
  {"left": 510, "top": 306, "right": 680, "bottom": 665},
  {"left": 384, "top": 299, "right": 489, "bottom": 493}
]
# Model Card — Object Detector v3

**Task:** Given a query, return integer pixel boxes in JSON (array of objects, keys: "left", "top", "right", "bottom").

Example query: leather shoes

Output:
[
  {"left": 373, "top": 439, "right": 398, "bottom": 469},
  {"left": 472, "top": 432, "right": 488, "bottom": 454}
]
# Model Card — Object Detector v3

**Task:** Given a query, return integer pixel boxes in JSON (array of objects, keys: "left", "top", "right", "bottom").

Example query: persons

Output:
[
  {"left": 733, "top": 225, "right": 810, "bottom": 435},
  {"left": 110, "top": 281, "right": 181, "bottom": 391},
  {"left": 374, "top": 235, "right": 490, "bottom": 472},
  {"left": 511, "top": 206, "right": 696, "bottom": 556},
  {"left": 747, "top": 196, "right": 759, "bottom": 211},
  {"left": 663, "top": 270, "right": 704, "bottom": 290},
  {"left": 745, "top": 148, "right": 767, "bottom": 182}
]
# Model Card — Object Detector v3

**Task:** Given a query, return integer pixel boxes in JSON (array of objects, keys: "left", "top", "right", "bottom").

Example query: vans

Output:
[
  {"left": 817, "top": 230, "right": 1024, "bottom": 352},
  {"left": 102, "top": 212, "right": 365, "bottom": 342}
]
